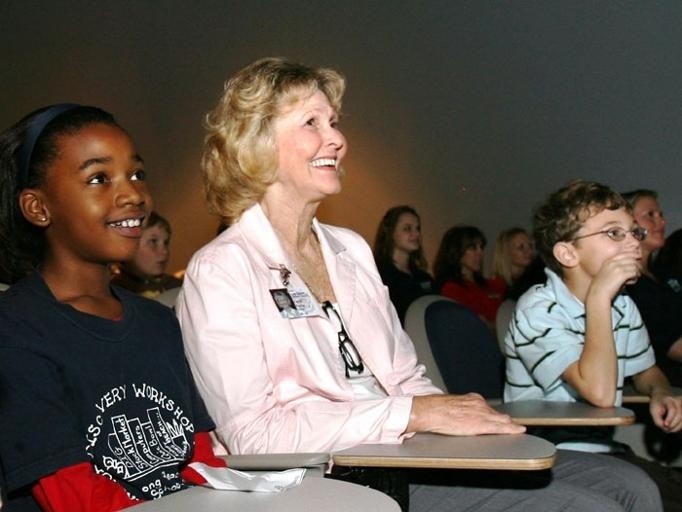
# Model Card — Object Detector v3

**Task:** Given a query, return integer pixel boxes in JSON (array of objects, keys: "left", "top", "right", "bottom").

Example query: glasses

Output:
[
  {"left": 566, "top": 226, "right": 648, "bottom": 241},
  {"left": 323, "top": 301, "right": 363, "bottom": 377}
]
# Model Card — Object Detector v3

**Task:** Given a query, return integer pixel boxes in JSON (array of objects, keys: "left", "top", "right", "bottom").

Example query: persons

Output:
[
  {"left": 0, "top": 101, "right": 231, "bottom": 512},
  {"left": 175, "top": 54, "right": 667, "bottom": 512},
  {"left": 618, "top": 187, "right": 682, "bottom": 397},
  {"left": 432, "top": 225, "right": 509, "bottom": 329},
  {"left": 371, "top": 204, "right": 440, "bottom": 328},
  {"left": 111, "top": 210, "right": 182, "bottom": 300},
  {"left": 501, "top": 181, "right": 682, "bottom": 512},
  {"left": 490, "top": 226, "right": 538, "bottom": 302}
]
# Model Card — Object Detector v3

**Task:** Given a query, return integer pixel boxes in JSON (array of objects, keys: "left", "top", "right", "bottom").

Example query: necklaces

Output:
[{"left": 272, "top": 230, "right": 329, "bottom": 305}]
